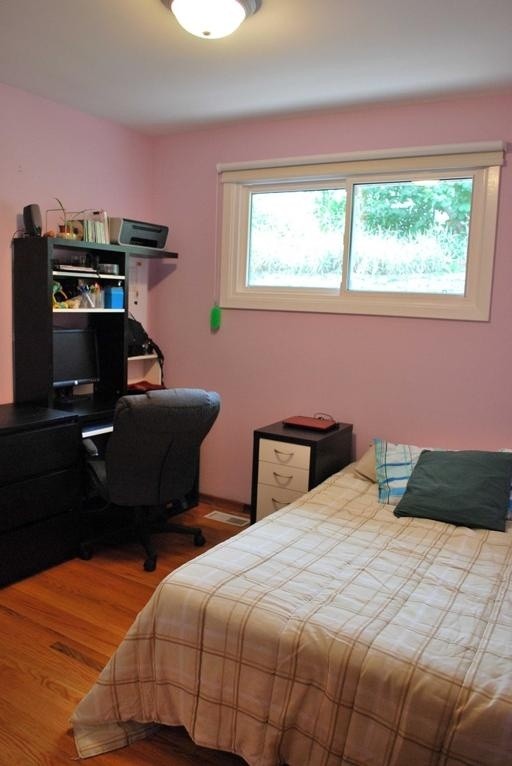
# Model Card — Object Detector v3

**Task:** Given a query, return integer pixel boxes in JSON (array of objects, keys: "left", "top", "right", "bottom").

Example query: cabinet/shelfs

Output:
[
  {"left": 10, "top": 240, "right": 179, "bottom": 405},
  {"left": 249, "top": 419, "right": 354, "bottom": 524}
]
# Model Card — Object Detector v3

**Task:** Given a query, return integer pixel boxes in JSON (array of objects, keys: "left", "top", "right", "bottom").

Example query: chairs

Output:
[{"left": 81, "top": 387, "right": 221, "bottom": 577}]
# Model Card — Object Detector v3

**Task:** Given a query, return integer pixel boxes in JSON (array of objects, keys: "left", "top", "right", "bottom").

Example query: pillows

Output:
[{"left": 356, "top": 435, "right": 512, "bottom": 532}]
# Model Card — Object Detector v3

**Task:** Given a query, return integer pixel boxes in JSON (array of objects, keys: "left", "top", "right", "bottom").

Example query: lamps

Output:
[{"left": 162, "top": 0, "right": 263, "bottom": 38}]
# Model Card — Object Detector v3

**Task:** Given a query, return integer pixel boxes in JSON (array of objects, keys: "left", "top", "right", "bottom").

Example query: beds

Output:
[{"left": 150, "top": 456, "right": 512, "bottom": 766}]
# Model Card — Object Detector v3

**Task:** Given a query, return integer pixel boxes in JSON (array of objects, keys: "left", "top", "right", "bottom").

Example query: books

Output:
[{"left": 77, "top": 219, "right": 106, "bottom": 245}]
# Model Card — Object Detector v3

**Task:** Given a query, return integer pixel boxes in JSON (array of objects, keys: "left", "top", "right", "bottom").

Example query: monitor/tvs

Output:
[{"left": 49, "top": 326, "right": 104, "bottom": 408}]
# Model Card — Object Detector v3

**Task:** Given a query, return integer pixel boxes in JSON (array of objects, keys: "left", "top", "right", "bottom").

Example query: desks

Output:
[{"left": 1, "top": 390, "right": 148, "bottom": 590}]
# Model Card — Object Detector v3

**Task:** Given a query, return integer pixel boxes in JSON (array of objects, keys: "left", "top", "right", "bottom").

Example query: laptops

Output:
[{"left": 281, "top": 415, "right": 337, "bottom": 433}]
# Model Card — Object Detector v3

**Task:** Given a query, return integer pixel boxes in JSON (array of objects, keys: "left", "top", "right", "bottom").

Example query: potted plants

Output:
[{"left": 54, "top": 196, "right": 95, "bottom": 240}]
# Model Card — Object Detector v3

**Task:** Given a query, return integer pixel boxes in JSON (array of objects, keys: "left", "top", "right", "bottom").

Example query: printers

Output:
[{"left": 108, "top": 215, "right": 169, "bottom": 251}]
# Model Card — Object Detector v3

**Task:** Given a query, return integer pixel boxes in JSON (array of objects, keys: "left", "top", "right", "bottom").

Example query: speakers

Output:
[{"left": 22, "top": 203, "right": 43, "bottom": 237}]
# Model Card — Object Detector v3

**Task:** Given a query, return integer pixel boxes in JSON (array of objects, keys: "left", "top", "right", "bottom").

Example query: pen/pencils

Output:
[{"left": 77, "top": 282, "right": 103, "bottom": 308}]
